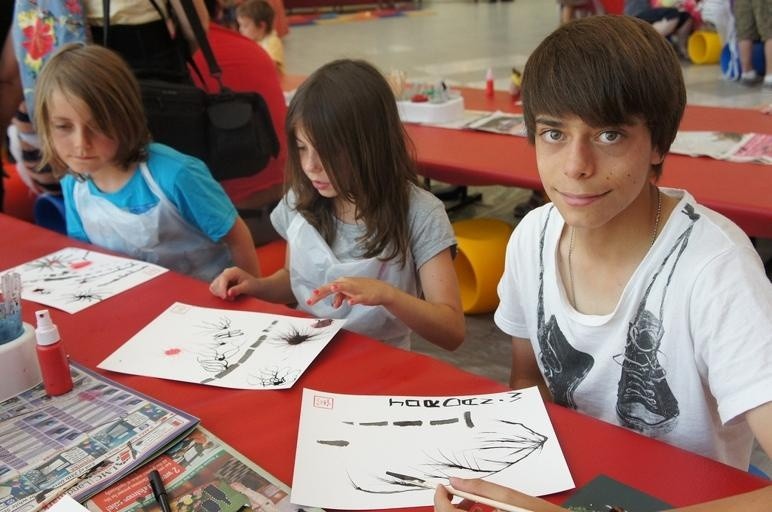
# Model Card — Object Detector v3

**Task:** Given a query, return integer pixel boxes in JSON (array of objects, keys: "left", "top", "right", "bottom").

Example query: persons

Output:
[
  {"left": 32, "top": 39, "right": 263, "bottom": 284},
  {"left": 431, "top": 9, "right": 772, "bottom": 511},
  {"left": 208, "top": 56, "right": 466, "bottom": 352},
  {"left": 1, "top": 1, "right": 298, "bottom": 255},
  {"left": 558, "top": 0, "right": 772, "bottom": 92}
]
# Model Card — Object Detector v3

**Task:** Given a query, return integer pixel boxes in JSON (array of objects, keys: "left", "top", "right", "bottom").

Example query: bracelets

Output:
[{"left": 607, "top": 504, "right": 628, "bottom": 512}]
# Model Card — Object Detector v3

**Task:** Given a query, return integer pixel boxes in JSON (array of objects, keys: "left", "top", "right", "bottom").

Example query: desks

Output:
[
  {"left": 1, "top": 217, "right": 772, "bottom": 511},
  {"left": 281, "top": 81, "right": 772, "bottom": 280}
]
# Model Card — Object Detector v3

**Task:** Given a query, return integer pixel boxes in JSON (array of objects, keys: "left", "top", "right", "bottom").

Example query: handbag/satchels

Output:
[
  {"left": 202, "top": 89, "right": 280, "bottom": 181},
  {"left": 137, "top": 78, "right": 207, "bottom": 157}
]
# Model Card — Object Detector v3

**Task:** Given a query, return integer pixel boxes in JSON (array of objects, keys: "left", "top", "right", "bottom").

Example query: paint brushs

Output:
[
  {"left": 26, "top": 460, "right": 104, "bottom": 512},
  {"left": 386, "top": 471, "right": 534, "bottom": 512}
]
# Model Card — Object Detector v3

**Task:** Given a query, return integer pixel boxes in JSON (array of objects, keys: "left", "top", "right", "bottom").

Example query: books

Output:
[
  {"left": 84, "top": 423, "right": 327, "bottom": 512},
  {"left": 0, "top": 356, "right": 203, "bottom": 512},
  {"left": 666, "top": 127, "right": 772, "bottom": 166},
  {"left": 560, "top": 474, "right": 675, "bottom": 511}
]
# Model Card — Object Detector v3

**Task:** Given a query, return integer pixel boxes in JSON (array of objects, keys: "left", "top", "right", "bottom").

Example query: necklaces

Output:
[{"left": 566, "top": 184, "right": 662, "bottom": 311}]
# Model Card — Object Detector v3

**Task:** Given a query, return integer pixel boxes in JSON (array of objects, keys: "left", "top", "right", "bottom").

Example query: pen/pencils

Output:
[{"left": 148, "top": 470, "right": 171, "bottom": 512}]
